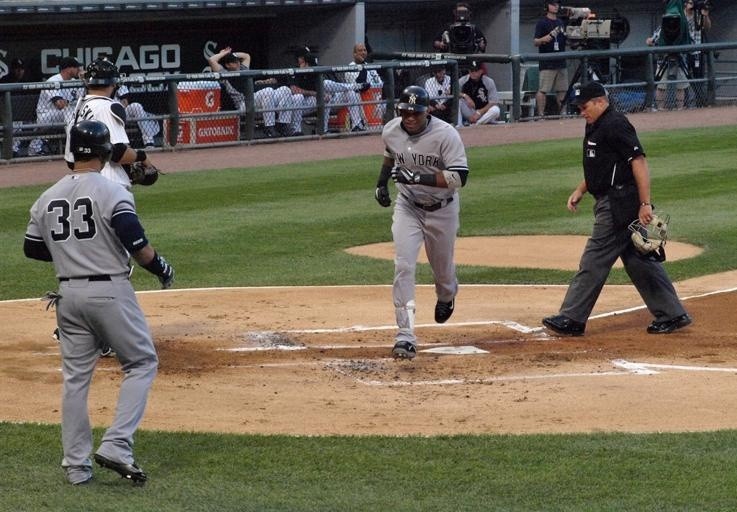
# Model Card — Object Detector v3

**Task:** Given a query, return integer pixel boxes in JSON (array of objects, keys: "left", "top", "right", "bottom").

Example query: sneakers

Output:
[
  {"left": 94, "top": 452, "right": 146, "bottom": 482},
  {"left": 66, "top": 464, "right": 93, "bottom": 486},
  {"left": 390, "top": 339, "right": 416, "bottom": 358},
  {"left": 542, "top": 313, "right": 584, "bottom": 336},
  {"left": 646, "top": 315, "right": 691, "bottom": 336},
  {"left": 433, "top": 298, "right": 455, "bottom": 323}
]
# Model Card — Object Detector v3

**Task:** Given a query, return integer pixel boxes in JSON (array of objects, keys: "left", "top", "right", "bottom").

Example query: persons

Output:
[
  {"left": 540, "top": 82, "right": 690, "bottom": 336},
  {"left": 684, "top": 1, "right": 711, "bottom": 108},
  {"left": 53, "top": 55, "right": 152, "bottom": 358},
  {"left": 533, "top": 2, "right": 568, "bottom": 119},
  {"left": 21, "top": 121, "right": 173, "bottom": 482},
  {"left": 374, "top": 85, "right": 467, "bottom": 357},
  {"left": 647, "top": 25, "right": 689, "bottom": 110}
]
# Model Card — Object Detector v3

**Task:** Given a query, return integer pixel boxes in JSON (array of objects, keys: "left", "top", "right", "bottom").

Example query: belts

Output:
[
  {"left": 57, "top": 274, "right": 113, "bottom": 283},
  {"left": 401, "top": 193, "right": 453, "bottom": 212}
]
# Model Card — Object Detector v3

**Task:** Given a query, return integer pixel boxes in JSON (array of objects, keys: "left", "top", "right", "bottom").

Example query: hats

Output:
[
  {"left": 569, "top": 81, "right": 606, "bottom": 107},
  {"left": 56, "top": 55, "right": 84, "bottom": 69},
  {"left": 467, "top": 58, "right": 481, "bottom": 73},
  {"left": 220, "top": 42, "right": 319, "bottom": 69}
]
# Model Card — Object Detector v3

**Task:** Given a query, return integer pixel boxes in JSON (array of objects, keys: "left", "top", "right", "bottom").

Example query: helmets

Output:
[
  {"left": 68, "top": 118, "right": 113, "bottom": 158},
  {"left": 397, "top": 86, "right": 429, "bottom": 114},
  {"left": 626, "top": 211, "right": 670, "bottom": 263},
  {"left": 132, "top": 163, "right": 159, "bottom": 186},
  {"left": 82, "top": 58, "right": 119, "bottom": 87}
]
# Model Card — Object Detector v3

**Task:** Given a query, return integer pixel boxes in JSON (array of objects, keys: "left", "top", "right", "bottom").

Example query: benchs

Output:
[{"left": 11, "top": 124, "right": 141, "bottom": 158}]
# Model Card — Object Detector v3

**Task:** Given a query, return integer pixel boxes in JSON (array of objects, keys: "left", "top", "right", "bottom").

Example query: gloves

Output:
[
  {"left": 159, "top": 266, "right": 175, "bottom": 289},
  {"left": 391, "top": 166, "right": 420, "bottom": 187},
  {"left": 375, "top": 185, "right": 392, "bottom": 207}
]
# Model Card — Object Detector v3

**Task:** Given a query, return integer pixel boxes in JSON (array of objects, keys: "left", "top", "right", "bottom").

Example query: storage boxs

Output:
[{"left": 177, "top": 81, "right": 221, "bottom": 115}]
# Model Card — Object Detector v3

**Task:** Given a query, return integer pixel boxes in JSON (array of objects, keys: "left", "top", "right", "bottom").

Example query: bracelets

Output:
[{"left": 639, "top": 202, "right": 650, "bottom": 206}]
